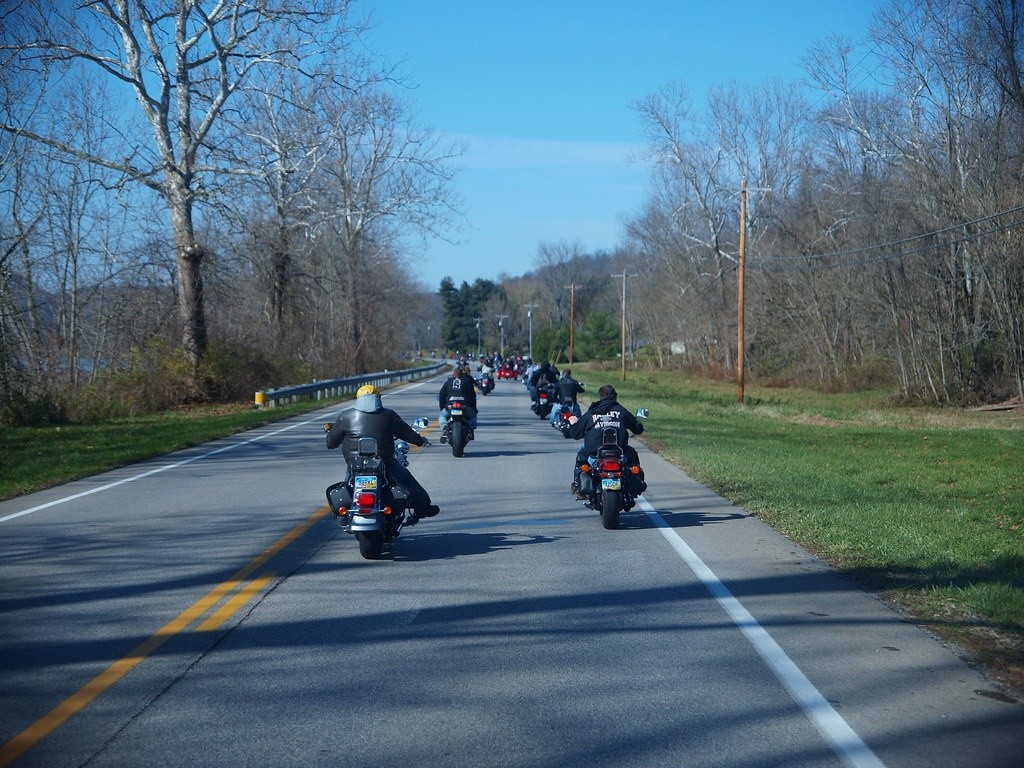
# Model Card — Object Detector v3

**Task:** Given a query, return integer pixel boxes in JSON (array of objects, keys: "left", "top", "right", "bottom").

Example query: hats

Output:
[{"left": 356, "top": 385, "right": 380, "bottom": 399}]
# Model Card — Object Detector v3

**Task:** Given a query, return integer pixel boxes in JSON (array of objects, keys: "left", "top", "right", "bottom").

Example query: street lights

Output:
[
  {"left": 473, "top": 316, "right": 484, "bottom": 356},
  {"left": 523, "top": 302, "right": 540, "bottom": 359},
  {"left": 610, "top": 268, "right": 640, "bottom": 381},
  {"left": 495, "top": 313, "right": 509, "bottom": 358},
  {"left": 564, "top": 282, "right": 582, "bottom": 364},
  {"left": 715, "top": 177, "right": 772, "bottom": 402}
]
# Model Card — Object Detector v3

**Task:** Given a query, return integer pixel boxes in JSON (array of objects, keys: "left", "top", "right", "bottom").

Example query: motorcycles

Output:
[
  {"left": 323, "top": 416, "right": 429, "bottom": 558},
  {"left": 457, "top": 354, "right": 476, "bottom": 362},
  {"left": 456, "top": 361, "right": 469, "bottom": 371},
  {"left": 545, "top": 382, "right": 586, "bottom": 433},
  {"left": 440, "top": 379, "right": 482, "bottom": 457},
  {"left": 515, "top": 359, "right": 561, "bottom": 389},
  {"left": 558, "top": 408, "right": 650, "bottom": 528},
  {"left": 528, "top": 380, "right": 560, "bottom": 420},
  {"left": 480, "top": 355, "right": 502, "bottom": 371},
  {"left": 475, "top": 366, "right": 497, "bottom": 395}
]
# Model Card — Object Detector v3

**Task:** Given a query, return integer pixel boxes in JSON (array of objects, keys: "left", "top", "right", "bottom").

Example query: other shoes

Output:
[
  {"left": 441, "top": 428, "right": 449, "bottom": 444},
  {"left": 414, "top": 505, "right": 440, "bottom": 518},
  {"left": 469, "top": 428, "right": 474, "bottom": 440},
  {"left": 340, "top": 518, "right": 351, "bottom": 528},
  {"left": 571, "top": 482, "right": 587, "bottom": 500}
]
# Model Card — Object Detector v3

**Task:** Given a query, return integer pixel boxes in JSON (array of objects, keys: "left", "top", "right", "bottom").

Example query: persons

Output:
[
  {"left": 442, "top": 369, "right": 479, "bottom": 438},
  {"left": 568, "top": 384, "right": 643, "bottom": 501},
  {"left": 403, "top": 349, "right": 560, "bottom": 406},
  {"left": 549, "top": 369, "right": 586, "bottom": 427},
  {"left": 328, "top": 385, "right": 440, "bottom": 527},
  {"left": 529, "top": 359, "right": 558, "bottom": 413}
]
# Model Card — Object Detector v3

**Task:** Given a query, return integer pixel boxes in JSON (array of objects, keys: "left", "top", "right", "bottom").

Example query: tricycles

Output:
[{"left": 497, "top": 365, "right": 518, "bottom": 380}]
